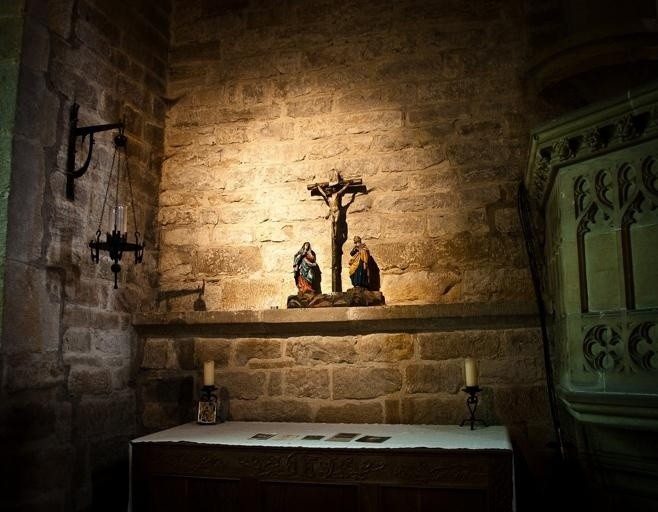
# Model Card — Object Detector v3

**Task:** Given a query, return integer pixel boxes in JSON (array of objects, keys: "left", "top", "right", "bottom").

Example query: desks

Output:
[{"left": 127, "top": 421, "right": 517, "bottom": 512}]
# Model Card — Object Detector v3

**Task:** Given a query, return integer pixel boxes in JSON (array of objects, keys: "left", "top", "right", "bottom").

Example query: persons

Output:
[
  {"left": 315, "top": 183, "right": 351, "bottom": 239},
  {"left": 347, "top": 235, "right": 372, "bottom": 286},
  {"left": 292, "top": 241, "right": 320, "bottom": 296}
]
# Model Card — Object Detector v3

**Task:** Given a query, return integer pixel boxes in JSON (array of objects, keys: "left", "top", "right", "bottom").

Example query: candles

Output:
[
  {"left": 464, "top": 355, "right": 479, "bottom": 386},
  {"left": 203, "top": 359, "right": 214, "bottom": 386},
  {"left": 110, "top": 206, "right": 127, "bottom": 237}
]
